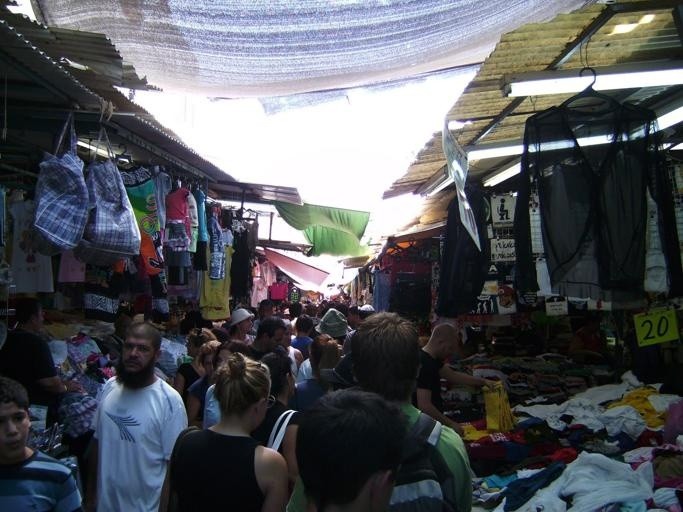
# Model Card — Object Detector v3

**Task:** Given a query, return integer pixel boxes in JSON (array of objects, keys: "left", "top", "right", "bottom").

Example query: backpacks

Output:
[{"left": 388, "top": 410, "right": 458, "bottom": 511}]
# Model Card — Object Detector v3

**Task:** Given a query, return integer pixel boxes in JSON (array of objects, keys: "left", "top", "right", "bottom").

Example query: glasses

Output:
[{"left": 266, "top": 395, "right": 276, "bottom": 409}]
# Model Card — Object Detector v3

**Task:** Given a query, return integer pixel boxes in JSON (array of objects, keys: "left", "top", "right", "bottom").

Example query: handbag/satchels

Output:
[{"left": 32, "top": 151, "right": 141, "bottom": 267}]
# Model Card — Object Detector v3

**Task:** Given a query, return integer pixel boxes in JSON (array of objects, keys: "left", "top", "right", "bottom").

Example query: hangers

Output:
[
  {"left": 525, "top": 67, "right": 657, "bottom": 124},
  {"left": 113, "top": 143, "right": 255, "bottom": 222}
]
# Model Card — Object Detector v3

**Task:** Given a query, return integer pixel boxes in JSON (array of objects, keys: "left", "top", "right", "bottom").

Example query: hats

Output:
[
  {"left": 314, "top": 308, "right": 352, "bottom": 337},
  {"left": 229, "top": 307, "right": 255, "bottom": 326}
]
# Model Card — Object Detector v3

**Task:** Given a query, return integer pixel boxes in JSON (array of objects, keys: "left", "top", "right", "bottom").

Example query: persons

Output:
[
  {"left": 625, "top": 317, "right": 665, "bottom": 385},
  {"left": 84, "top": 291, "right": 492, "bottom": 511},
  {"left": 1, "top": 298, "right": 84, "bottom": 511}
]
